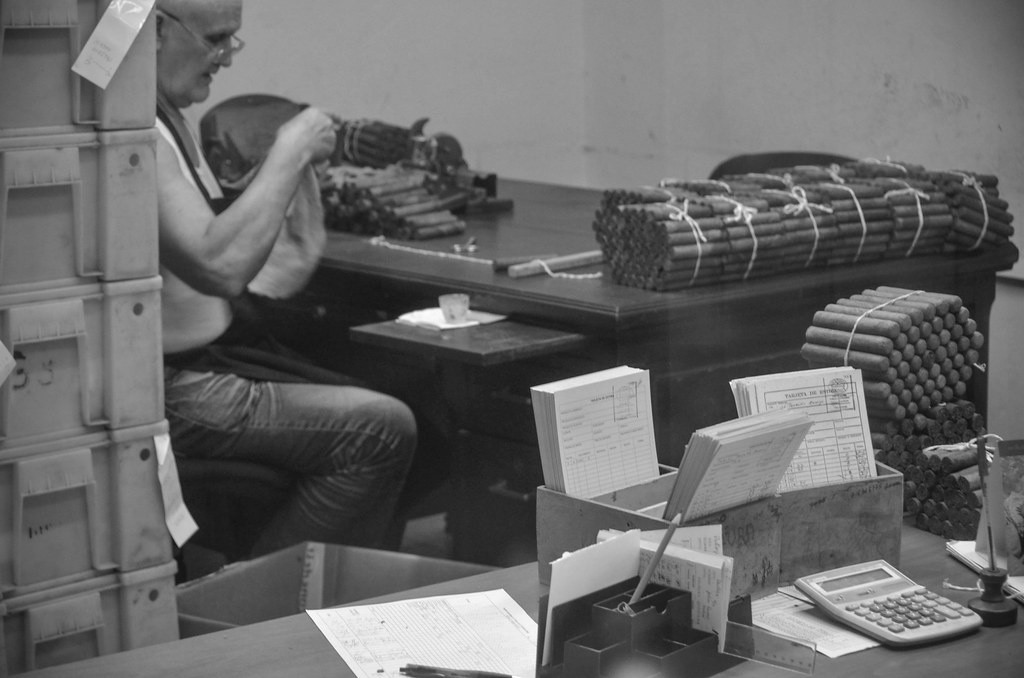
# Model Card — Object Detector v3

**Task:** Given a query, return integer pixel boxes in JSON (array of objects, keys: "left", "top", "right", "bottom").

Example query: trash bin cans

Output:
[{"left": 173, "top": 541, "right": 511, "bottom": 640}]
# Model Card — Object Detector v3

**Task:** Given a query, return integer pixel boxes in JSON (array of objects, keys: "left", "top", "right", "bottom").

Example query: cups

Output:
[{"left": 438, "top": 293, "right": 470, "bottom": 324}]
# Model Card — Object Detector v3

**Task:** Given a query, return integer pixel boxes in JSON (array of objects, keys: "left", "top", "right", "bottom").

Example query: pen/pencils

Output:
[
  {"left": 400, "top": 663, "right": 512, "bottom": 678},
  {"left": 627, "top": 510, "right": 684, "bottom": 605}
]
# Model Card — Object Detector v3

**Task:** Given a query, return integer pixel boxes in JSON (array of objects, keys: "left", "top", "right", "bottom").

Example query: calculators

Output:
[{"left": 792, "top": 559, "right": 984, "bottom": 649}]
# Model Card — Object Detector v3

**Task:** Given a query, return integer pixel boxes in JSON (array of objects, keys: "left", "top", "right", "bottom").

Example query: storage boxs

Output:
[
  {"left": 177, "top": 541, "right": 505, "bottom": 640},
  {"left": 781, "top": 460, "right": 903, "bottom": 587},
  {"left": 0, "top": 0, "right": 183, "bottom": 678},
  {"left": 537, "top": 464, "right": 781, "bottom": 612}
]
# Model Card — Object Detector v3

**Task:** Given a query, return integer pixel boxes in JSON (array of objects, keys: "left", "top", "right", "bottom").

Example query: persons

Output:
[{"left": 156, "top": 0, "right": 415, "bottom": 555}]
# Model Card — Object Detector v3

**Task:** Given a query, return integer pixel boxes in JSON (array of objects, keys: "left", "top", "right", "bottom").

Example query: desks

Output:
[
  {"left": 0, "top": 524, "right": 1024, "bottom": 678},
  {"left": 217, "top": 177, "right": 1019, "bottom": 567}
]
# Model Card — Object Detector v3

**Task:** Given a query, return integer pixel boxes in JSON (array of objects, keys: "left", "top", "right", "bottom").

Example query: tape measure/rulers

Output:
[{"left": 724, "top": 620, "right": 816, "bottom": 676}]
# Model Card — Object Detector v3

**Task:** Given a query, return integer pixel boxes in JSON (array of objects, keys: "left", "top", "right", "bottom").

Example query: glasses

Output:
[{"left": 157, "top": 7, "right": 245, "bottom": 65}]
